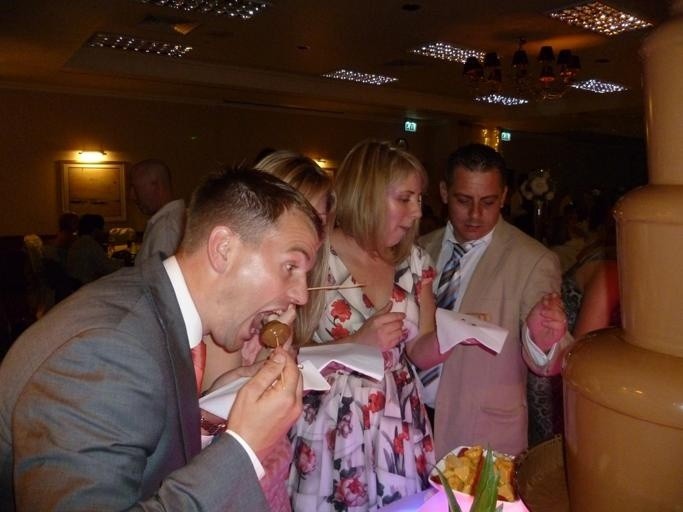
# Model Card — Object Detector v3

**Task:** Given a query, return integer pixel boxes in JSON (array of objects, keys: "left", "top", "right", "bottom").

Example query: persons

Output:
[
  {"left": 527, "top": 235, "right": 621, "bottom": 448},
  {"left": 289, "top": 139, "right": 493, "bottom": 512},
  {"left": 66, "top": 214, "right": 122, "bottom": 283},
  {"left": 414, "top": 143, "right": 570, "bottom": 465},
  {"left": 0, "top": 160, "right": 330, "bottom": 512},
  {"left": 129, "top": 159, "right": 189, "bottom": 267},
  {"left": 201, "top": 149, "right": 338, "bottom": 512}
]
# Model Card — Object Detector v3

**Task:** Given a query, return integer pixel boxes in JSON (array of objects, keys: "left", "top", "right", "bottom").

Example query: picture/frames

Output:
[{"left": 56, "top": 159, "right": 130, "bottom": 225}]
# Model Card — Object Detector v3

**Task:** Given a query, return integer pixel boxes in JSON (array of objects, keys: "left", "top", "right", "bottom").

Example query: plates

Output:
[{"left": 429, "top": 445, "right": 525, "bottom": 505}]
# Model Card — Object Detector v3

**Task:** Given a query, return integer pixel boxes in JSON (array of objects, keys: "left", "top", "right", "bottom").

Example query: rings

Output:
[{"left": 562, "top": 319, "right": 566, "bottom": 325}]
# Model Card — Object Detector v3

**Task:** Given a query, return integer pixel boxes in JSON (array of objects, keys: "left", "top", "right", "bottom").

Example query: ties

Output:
[{"left": 415, "top": 243, "right": 473, "bottom": 386}]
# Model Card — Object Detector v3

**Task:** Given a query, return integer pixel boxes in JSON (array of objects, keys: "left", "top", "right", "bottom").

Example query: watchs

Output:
[{"left": 200, "top": 416, "right": 228, "bottom": 435}]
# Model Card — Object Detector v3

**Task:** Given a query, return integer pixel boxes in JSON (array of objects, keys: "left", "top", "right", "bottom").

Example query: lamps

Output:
[{"left": 462, "top": 39, "right": 582, "bottom": 100}]
